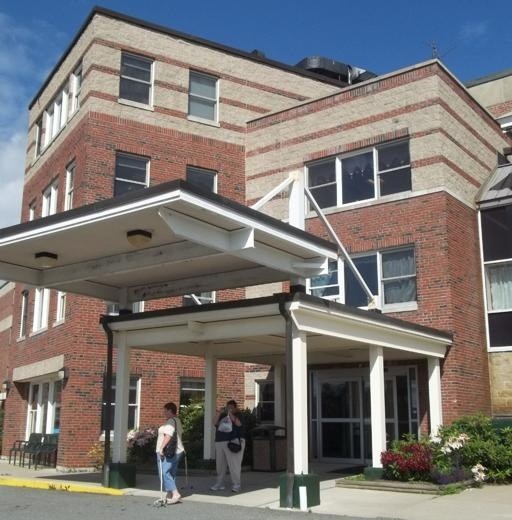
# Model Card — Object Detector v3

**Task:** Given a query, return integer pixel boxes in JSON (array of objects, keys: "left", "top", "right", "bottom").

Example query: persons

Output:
[
  {"left": 209, "top": 399, "right": 247, "bottom": 492},
  {"left": 155, "top": 401, "right": 187, "bottom": 505}
]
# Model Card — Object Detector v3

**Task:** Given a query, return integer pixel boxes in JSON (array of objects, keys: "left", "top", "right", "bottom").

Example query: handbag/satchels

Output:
[
  {"left": 227, "top": 415, "right": 241, "bottom": 453},
  {"left": 163, "top": 416, "right": 177, "bottom": 458}
]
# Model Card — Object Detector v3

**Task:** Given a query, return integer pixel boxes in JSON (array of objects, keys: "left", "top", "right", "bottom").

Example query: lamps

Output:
[
  {"left": 34, "top": 250, "right": 59, "bottom": 270},
  {"left": 127, "top": 229, "right": 153, "bottom": 246}
]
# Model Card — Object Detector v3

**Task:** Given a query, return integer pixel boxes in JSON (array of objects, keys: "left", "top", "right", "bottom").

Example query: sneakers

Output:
[
  {"left": 231, "top": 486, "right": 242, "bottom": 492},
  {"left": 209, "top": 484, "right": 225, "bottom": 491}
]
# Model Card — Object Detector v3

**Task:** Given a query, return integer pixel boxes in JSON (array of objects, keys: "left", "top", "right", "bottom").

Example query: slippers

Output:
[{"left": 167, "top": 499, "right": 183, "bottom": 504}]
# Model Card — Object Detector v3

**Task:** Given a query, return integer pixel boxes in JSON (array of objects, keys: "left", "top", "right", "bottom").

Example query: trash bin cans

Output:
[{"left": 250, "top": 424, "right": 288, "bottom": 473}]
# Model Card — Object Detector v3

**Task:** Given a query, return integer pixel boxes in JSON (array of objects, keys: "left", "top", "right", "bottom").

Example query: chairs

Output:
[{"left": 8, "top": 432, "right": 59, "bottom": 471}]
